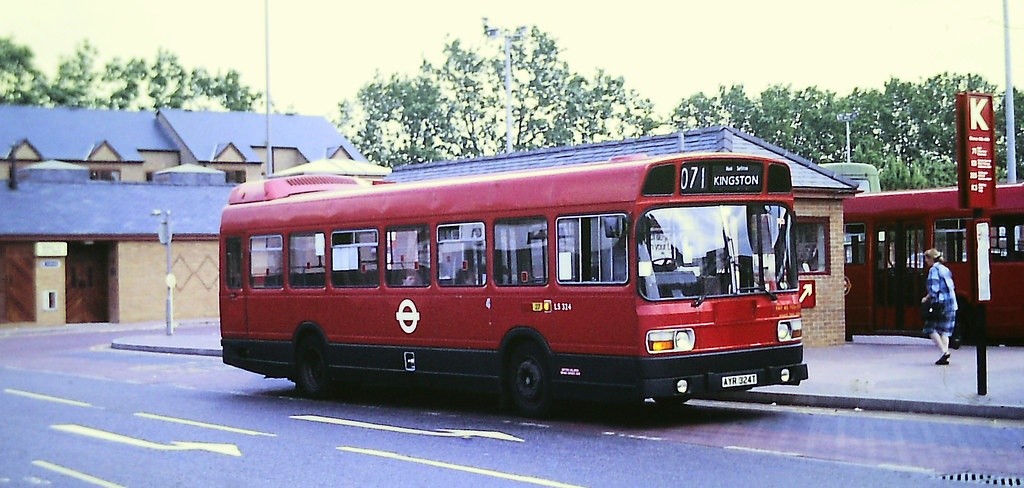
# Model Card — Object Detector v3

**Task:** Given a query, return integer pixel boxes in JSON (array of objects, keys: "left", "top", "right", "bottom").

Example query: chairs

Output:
[
  {"left": 302, "top": 265, "right": 326, "bottom": 286},
  {"left": 332, "top": 270, "right": 363, "bottom": 285},
  {"left": 391, "top": 262, "right": 419, "bottom": 285},
  {"left": 274, "top": 266, "right": 303, "bottom": 286},
  {"left": 359, "top": 261, "right": 378, "bottom": 285}
]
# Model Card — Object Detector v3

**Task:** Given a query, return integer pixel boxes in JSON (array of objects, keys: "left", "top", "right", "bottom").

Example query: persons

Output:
[{"left": 921, "top": 249, "right": 958, "bottom": 365}]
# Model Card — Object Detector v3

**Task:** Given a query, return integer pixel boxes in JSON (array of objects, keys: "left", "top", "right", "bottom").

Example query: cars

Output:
[{"left": 907, "top": 252, "right": 924, "bottom": 269}]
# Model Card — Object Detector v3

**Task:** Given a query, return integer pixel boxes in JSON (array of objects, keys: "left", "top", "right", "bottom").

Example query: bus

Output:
[
  {"left": 219, "top": 150, "right": 810, "bottom": 422},
  {"left": 817, "top": 162, "right": 888, "bottom": 270}
]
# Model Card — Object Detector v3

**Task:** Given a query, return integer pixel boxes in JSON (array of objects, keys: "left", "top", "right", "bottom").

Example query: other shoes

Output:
[
  {"left": 938, "top": 352, "right": 951, "bottom": 363},
  {"left": 935, "top": 360, "right": 949, "bottom": 365}
]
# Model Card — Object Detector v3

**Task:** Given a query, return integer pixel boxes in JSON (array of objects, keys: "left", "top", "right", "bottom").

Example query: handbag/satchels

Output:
[{"left": 924, "top": 302, "right": 945, "bottom": 320}]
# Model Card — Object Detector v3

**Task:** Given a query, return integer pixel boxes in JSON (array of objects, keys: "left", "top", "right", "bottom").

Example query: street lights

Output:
[
  {"left": 484, "top": 26, "right": 529, "bottom": 153},
  {"left": 837, "top": 111, "right": 860, "bottom": 163},
  {"left": 151, "top": 208, "right": 173, "bottom": 337}
]
